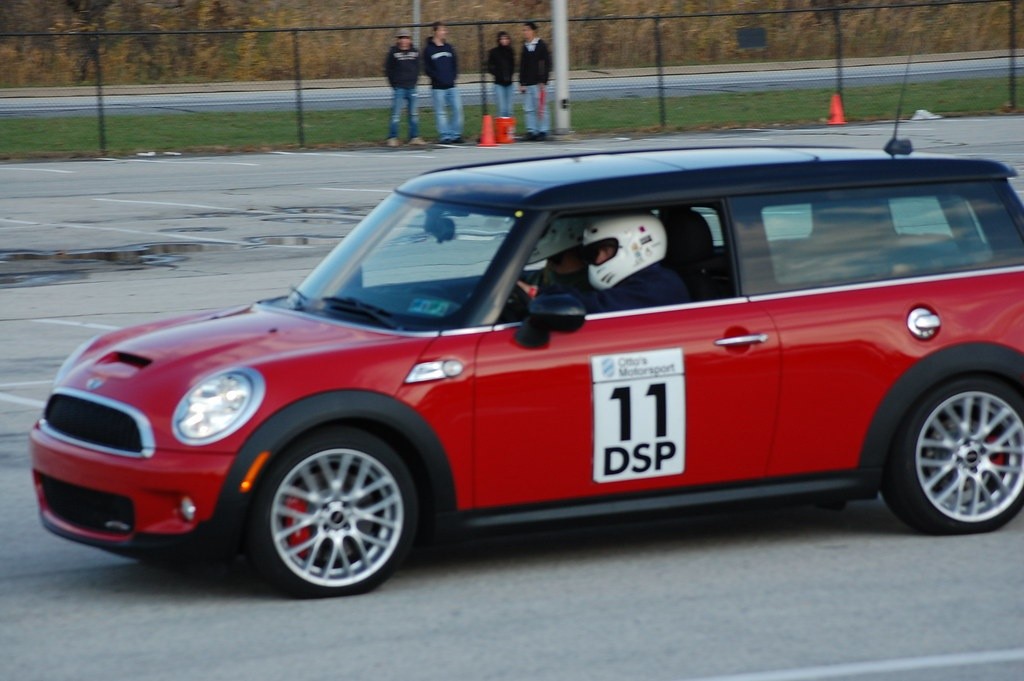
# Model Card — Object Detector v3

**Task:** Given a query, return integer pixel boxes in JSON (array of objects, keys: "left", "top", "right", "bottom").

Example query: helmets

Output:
[
  {"left": 582, "top": 216, "right": 668, "bottom": 291},
  {"left": 524, "top": 217, "right": 587, "bottom": 266}
]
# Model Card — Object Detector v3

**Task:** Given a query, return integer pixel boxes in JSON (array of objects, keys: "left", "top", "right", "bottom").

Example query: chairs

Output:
[{"left": 661, "top": 208, "right": 725, "bottom": 298}]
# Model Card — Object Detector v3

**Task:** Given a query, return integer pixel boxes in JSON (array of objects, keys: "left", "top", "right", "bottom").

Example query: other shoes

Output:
[
  {"left": 388, "top": 136, "right": 401, "bottom": 148},
  {"left": 523, "top": 129, "right": 552, "bottom": 143},
  {"left": 409, "top": 136, "right": 428, "bottom": 145},
  {"left": 440, "top": 136, "right": 467, "bottom": 143}
]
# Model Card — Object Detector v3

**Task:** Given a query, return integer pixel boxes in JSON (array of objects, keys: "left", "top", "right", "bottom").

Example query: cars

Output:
[{"left": 23, "top": 125, "right": 1023, "bottom": 600}]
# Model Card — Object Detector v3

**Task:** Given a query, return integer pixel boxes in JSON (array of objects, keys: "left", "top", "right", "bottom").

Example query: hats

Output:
[{"left": 394, "top": 28, "right": 412, "bottom": 38}]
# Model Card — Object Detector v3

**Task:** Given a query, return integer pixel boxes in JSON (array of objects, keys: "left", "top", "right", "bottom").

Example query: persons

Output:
[
  {"left": 449, "top": 211, "right": 690, "bottom": 322},
  {"left": 384, "top": 21, "right": 552, "bottom": 147}
]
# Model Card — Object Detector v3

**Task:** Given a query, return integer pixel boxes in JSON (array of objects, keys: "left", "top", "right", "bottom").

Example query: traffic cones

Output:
[
  {"left": 475, "top": 115, "right": 500, "bottom": 147},
  {"left": 826, "top": 93, "right": 847, "bottom": 126}
]
